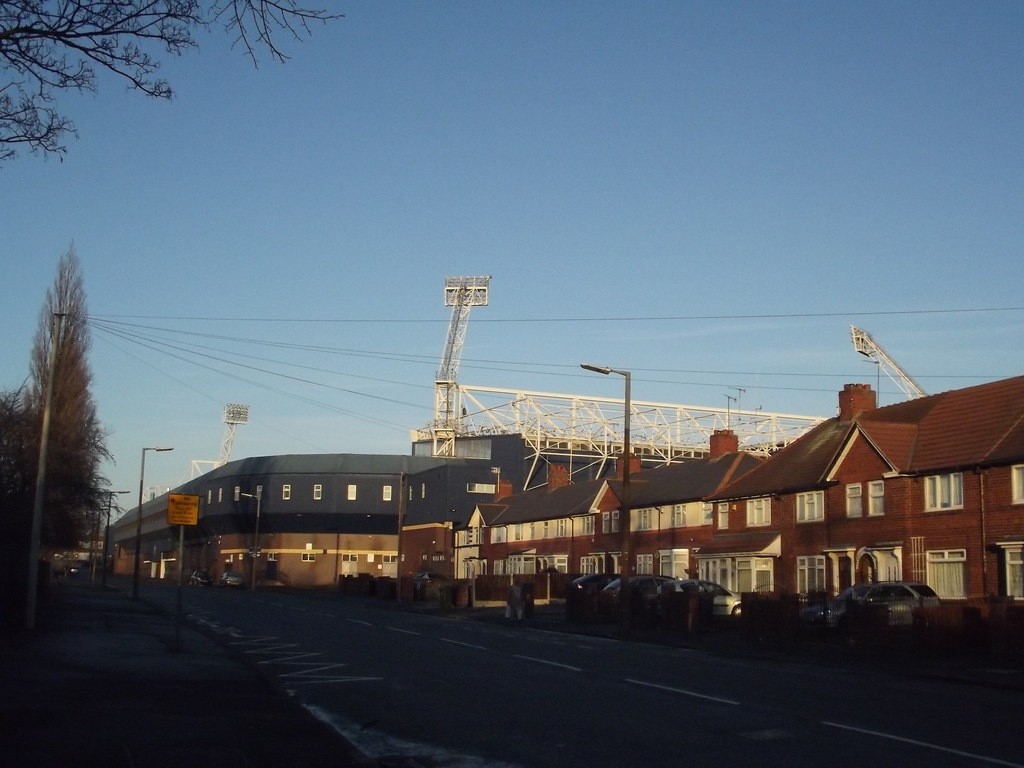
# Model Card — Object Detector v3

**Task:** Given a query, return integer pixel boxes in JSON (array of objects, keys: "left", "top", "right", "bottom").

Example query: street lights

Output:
[
  {"left": 580, "top": 362, "right": 633, "bottom": 641},
  {"left": 133, "top": 448, "right": 174, "bottom": 593},
  {"left": 102, "top": 490, "right": 131, "bottom": 590},
  {"left": 240, "top": 492, "right": 260, "bottom": 590}
]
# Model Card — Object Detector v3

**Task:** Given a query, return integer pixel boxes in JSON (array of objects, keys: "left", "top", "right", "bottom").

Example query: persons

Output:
[
  {"left": 505, "top": 578, "right": 525, "bottom": 622},
  {"left": 447, "top": 580, "right": 462, "bottom": 608}
]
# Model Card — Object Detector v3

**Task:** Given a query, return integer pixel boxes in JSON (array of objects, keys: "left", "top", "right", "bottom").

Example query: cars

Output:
[
  {"left": 824, "top": 584, "right": 943, "bottom": 641},
  {"left": 219, "top": 571, "right": 242, "bottom": 588},
  {"left": 414, "top": 571, "right": 450, "bottom": 601},
  {"left": 566, "top": 573, "right": 633, "bottom": 615},
  {"left": 594, "top": 574, "right": 678, "bottom": 615},
  {"left": 190, "top": 570, "right": 210, "bottom": 586},
  {"left": 636, "top": 579, "right": 742, "bottom": 618}
]
